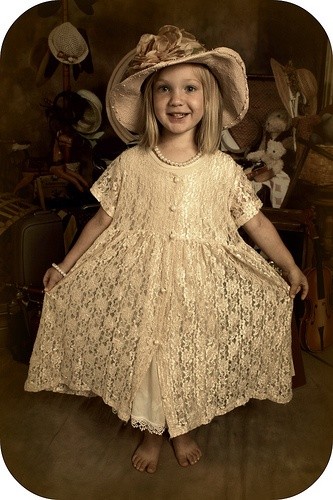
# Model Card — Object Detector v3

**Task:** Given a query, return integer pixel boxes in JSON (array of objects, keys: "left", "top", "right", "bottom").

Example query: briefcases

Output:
[{"left": 9, "top": 208, "right": 71, "bottom": 290}]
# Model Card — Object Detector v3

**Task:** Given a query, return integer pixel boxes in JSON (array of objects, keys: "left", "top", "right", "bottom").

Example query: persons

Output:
[{"left": 22, "top": 26, "right": 308, "bottom": 473}]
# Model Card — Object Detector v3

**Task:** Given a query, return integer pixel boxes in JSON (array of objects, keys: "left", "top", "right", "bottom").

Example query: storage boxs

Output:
[{"left": 10, "top": 212, "right": 64, "bottom": 297}]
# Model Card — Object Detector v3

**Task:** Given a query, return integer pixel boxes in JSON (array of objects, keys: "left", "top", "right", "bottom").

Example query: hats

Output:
[
  {"left": 108, "top": 25, "right": 249, "bottom": 135},
  {"left": 47, "top": 22, "right": 89, "bottom": 66},
  {"left": 269, "top": 56, "right": 318, "bottom": 118},
  {"left": 70, "top": 88, "right": 103, "bottom": 134},
  {"left": 106, "top": 48, "right": 137, "bottom": 146}
]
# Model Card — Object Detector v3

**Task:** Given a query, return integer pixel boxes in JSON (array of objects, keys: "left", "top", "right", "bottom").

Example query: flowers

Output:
[{"left": 128, "top": 25, "right": 207, "bottom": 70}]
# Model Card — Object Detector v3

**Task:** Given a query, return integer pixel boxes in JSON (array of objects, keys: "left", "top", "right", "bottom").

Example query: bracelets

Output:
[{"left": 51, "top": 262, "right": 65, "bottom": 277}]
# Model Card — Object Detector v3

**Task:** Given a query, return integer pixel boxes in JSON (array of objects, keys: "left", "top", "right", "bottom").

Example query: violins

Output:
[{"left": 299, "top": 209, "right": 333, "bottom": 352}]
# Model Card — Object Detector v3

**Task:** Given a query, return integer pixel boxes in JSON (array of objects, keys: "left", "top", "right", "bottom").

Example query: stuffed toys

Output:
[
  {"left": 243, "top": 140, "right": 286, "bottom": 196},
  {"left": 265, "top": 111, "right": 292, "bottom": 140}
]
